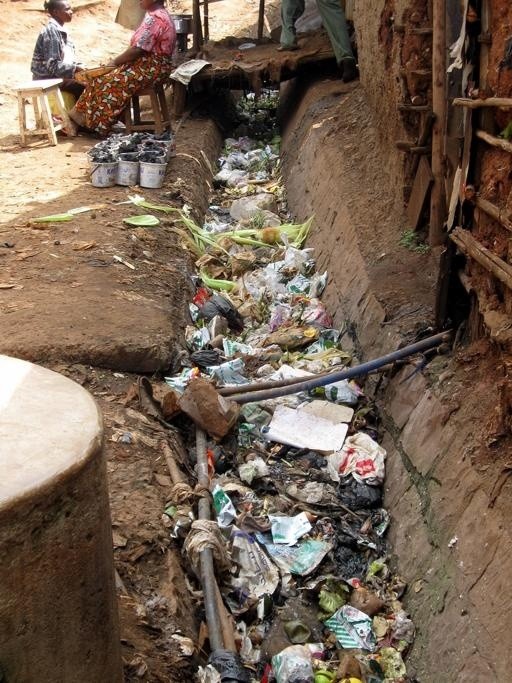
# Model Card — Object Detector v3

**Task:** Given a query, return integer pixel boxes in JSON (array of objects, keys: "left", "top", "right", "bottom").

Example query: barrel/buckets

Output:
[{"left": 87, "top": 132, "right": 175, "bottom": 188}]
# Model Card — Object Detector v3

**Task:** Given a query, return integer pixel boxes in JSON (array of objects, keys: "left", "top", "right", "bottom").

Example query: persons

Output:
[
  {"left": 31, "top": 0, "right": 89, "bottom": 104},
  {"left": 277, "top": 0, "right": 358, "bottom": 82},
  {"left": 52, "top": 0, "right": 176, "bottom": 136}
]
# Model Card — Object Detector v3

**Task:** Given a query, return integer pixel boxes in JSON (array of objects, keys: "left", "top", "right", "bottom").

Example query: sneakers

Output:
[
  {"left": 342, "top": 58, "right": 359, "bottom": 83},
  {"left": 275, "top": 46, "right": 297, "bottom": 51}
]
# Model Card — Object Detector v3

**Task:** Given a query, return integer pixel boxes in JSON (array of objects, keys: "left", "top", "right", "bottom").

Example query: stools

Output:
[
  {"left": 124, "top": 80, "right": 173, "bottom": 138},
  {"left": 11, "top": 77, "right": 73, "bottom": 146}
]
